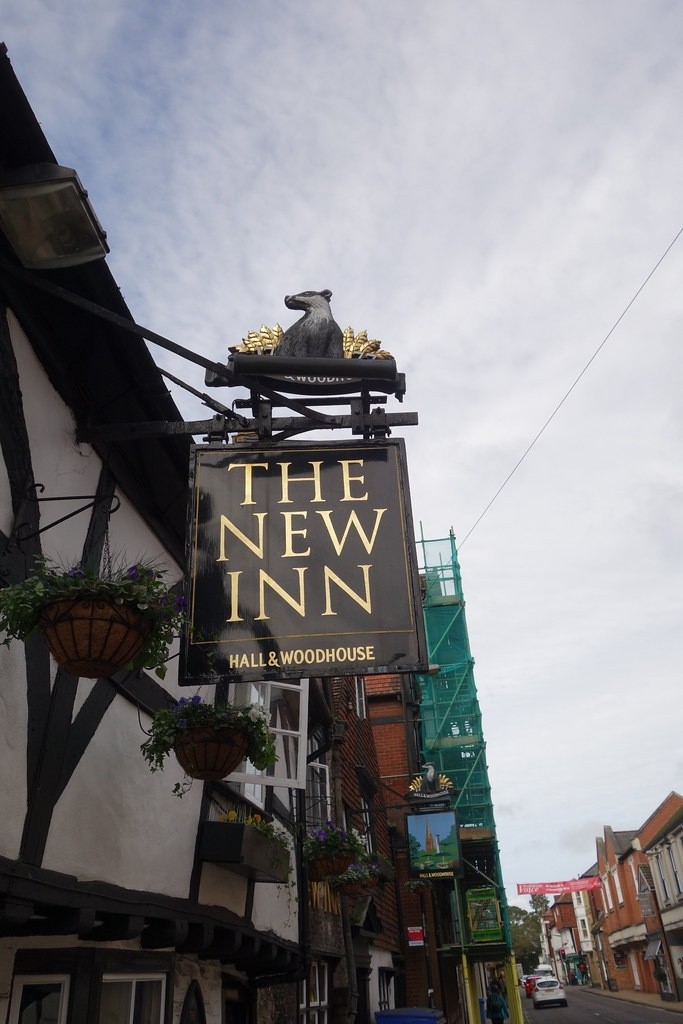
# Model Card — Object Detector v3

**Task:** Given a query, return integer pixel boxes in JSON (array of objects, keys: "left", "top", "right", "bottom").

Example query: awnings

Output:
[{"left": 645, "top": 939, "right": 664, "bottom": 956}]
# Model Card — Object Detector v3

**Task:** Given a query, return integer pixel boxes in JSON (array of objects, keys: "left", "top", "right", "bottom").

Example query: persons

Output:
[
  {"left": 569, "top": 970, "right": 576, "bottom": 986},
  {"left": 486, "top": 978, "right": 509, "bottom": 1024}
]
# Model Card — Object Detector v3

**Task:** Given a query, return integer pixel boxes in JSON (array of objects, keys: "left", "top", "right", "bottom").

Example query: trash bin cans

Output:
[
  {"left": 374, "top": 1008, "right": 446, "bottom": 1024},
  {"left": 608, "top": 979, "right": 618, "bottom": 992},
  {"left": 479, "top": 997, "right": 486, "bottom": 1024}
]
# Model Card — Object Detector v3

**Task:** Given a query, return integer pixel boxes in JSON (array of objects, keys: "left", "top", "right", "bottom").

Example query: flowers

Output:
[
  {"left": 216, "top": 812, "right": 300, "bottom": 851},
  {"left": 404, "top": 878, "right": 432, "bottom": 890},
  {"left": 305, "top": 821, "right": 366, "bottom": 858},
  {"left": 145, "top": 696, "right": 278, "bottom": 798},
  {"left": 347, "top": 855, "right": 399, "bottom": 878},
  {"left": 0, "top": 556, "right": 188, "bottom": 676}
]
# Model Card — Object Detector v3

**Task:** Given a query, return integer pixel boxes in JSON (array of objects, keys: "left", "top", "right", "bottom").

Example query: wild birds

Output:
[{"left": 421, "top": 762, "right": 441, "bottom": 794}]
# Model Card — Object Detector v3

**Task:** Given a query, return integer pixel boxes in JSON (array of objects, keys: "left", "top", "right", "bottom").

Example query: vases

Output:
[
  {"left": 203, "top": 824, "right": 289, "bottom": 886},
  {"left": 313, "top": 847, "right": 355, "bottom": 879},
  {"left": 43, "top": 594, "right": 156, "bottom": 680},
  {"left": 412, "top": 885, "right": 430, "bottom": 894},
  {"left": 343, "top": 874, "right": 377, "bottom": 898},
  {"left": 172, "top": 725, "right": 252, "bottom": 783}
]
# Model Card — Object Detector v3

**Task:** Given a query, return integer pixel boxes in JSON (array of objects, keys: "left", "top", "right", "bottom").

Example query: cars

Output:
[
  {"left": 520, "top": 975, "right": 529, "bottom": 987},
  {"left": 523, "top": 977, "right": 539, "bottom": 994},
  {"left": 533, "top": 969, "right": 555, "bottom": 979},
  {"left": 531, "top": 979, "right": 567, "bottom": 1008}
]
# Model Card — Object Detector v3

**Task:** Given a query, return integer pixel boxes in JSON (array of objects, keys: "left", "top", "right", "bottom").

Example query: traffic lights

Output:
[{"left": 560, "top": 949, "right": 565, "bottom": 959}]
